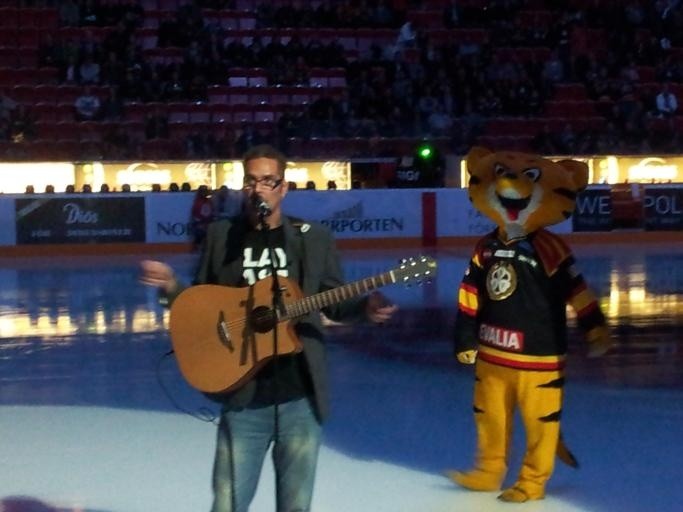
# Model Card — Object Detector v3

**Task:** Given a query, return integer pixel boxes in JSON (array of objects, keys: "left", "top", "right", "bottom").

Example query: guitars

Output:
[{"left": 169, "top": 254, "right": 437, "bottom": 399}]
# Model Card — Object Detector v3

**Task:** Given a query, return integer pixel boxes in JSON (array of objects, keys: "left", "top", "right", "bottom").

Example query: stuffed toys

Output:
[{"left": 443, "top": 147, "right": 613, "bottom": 504}]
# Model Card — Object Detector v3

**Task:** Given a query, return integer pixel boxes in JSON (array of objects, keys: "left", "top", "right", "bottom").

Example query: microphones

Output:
[{"left": 250, "top": 194, "right": 271, "bottom": 217}]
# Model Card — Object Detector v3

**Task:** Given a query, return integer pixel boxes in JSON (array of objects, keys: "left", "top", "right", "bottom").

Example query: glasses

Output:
[{"left": 242, "top": 173, "right": 282, "bottom": 190}]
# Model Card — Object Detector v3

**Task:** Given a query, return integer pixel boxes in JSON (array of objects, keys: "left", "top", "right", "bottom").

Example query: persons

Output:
[
  {"left": 140, "top": 143, "right": 398, "bottom": 511},
  {"left": 0, "top": 1, "right": 682, "bottom": 195}
]
